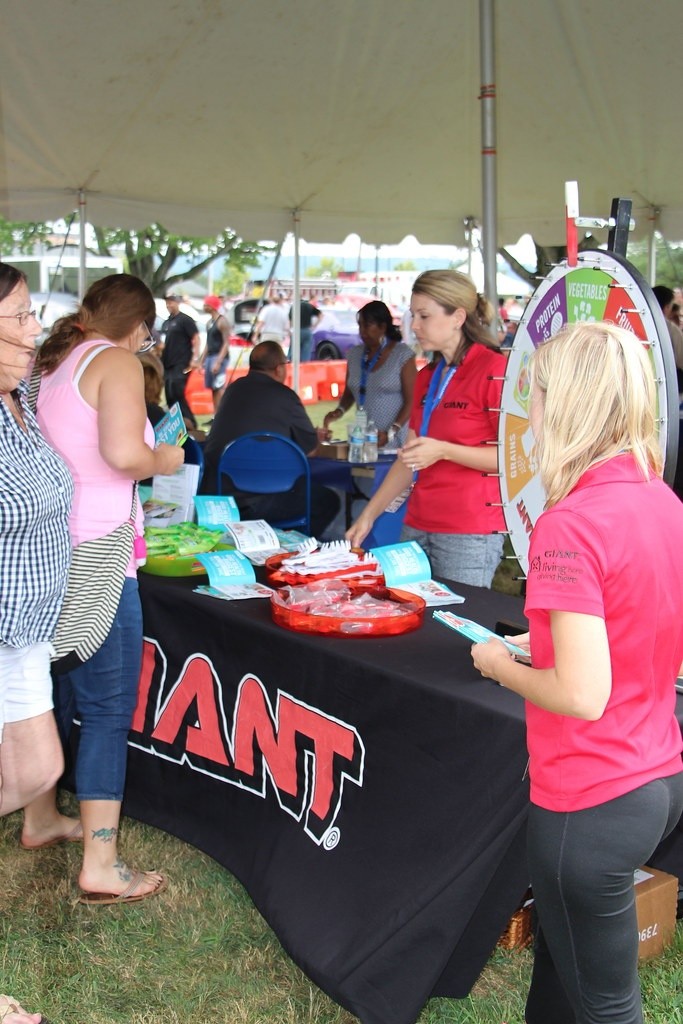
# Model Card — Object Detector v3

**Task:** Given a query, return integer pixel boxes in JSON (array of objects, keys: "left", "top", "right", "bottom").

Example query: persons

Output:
[
  {"left": 131, "top": 271, "right": 518, "bottom": 590},
  {"left": 20, "top": 273, "right": 185, "bottom": 899},
  {"left": 471, "top": 323, "right": 682, "bottom": 1024},
  {"left": 0, "top": 259, "right": 68, "bottom": 822},
  {"left": 646, "top": 282, "right": 683, "bottom": 390}
]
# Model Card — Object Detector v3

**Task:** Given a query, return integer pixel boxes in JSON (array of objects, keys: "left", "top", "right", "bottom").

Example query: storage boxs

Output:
[{"left": 632, "top": 865, "right": 679, "bottom": 964}]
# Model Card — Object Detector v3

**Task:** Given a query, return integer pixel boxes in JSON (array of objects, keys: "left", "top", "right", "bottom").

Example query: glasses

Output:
[
  {"left": 138, "top": 320, "right": 156, "bottom": 353},
  {"left": 0, "top": 310, "right": 36, "bottom": 326}
]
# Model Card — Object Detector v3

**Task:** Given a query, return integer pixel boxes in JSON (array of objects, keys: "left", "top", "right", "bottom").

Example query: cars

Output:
[{"left": 30, "top": 295, "right": 365, "bottom": 367}]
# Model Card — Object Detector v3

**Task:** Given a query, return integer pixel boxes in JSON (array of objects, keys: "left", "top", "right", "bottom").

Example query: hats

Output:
[
  {"left": 202, "top": 295, "right": 222, "bottom": 311},
  {"left": 163, "top": 295, "right": 180, "bottom": 304}
]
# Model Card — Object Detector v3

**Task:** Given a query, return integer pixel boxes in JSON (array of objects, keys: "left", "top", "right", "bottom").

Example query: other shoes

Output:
[{"left": 201, "top": 418, "right": 214, "bottom": 426}]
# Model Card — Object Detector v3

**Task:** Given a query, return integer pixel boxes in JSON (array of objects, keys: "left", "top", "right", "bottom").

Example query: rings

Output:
[{"left": 410, "top": 464, "right": 416, "bottom": 473}]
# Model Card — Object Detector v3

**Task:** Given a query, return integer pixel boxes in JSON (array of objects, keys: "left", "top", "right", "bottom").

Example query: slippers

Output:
[
  {"left": 78, "top": 873, "right": 169, "bottom": 905},
  {"left": 0, "top": 994, "right": 48, "bottom": 1024},
  {"left": 19, "top": 817, "right": 83, "bottom": 850}
]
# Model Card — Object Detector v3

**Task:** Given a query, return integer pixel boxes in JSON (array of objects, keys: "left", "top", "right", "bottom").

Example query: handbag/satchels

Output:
[{"left": 50, "top": 519, "right": 135, "bottom": 676}]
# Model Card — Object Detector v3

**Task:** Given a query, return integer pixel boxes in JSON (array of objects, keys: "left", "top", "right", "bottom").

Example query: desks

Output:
[
  {"left": 132, "top": 529, "right": 683, "bottom": 1024},
  {"left": 300, "top": 451, "right": 419, "bottom": 552}
]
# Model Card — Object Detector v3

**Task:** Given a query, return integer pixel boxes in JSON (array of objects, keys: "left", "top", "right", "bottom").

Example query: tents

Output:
[{"left": 0, "top": 0, "right": 683, "bottom": 405}]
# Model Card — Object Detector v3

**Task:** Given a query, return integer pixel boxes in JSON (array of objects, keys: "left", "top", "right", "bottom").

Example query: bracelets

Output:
[
  {"left": 337, "top": 406, "right": 345, "bottom": 413},
  {"left": 392, "top": 422, "right": 401, "bottom": 429}
]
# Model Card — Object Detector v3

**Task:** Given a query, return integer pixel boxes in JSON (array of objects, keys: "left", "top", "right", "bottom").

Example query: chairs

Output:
[{"left": 217, "top": 431, "right": 312, "bottom": 535}]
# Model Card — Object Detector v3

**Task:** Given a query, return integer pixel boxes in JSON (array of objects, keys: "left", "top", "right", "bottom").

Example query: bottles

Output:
[
  {"left": 347, "top": 428, "right": 364, "bottom": 463},
  {"left": 364, "top": 421, "right": 378, "bottom": 462}
]
